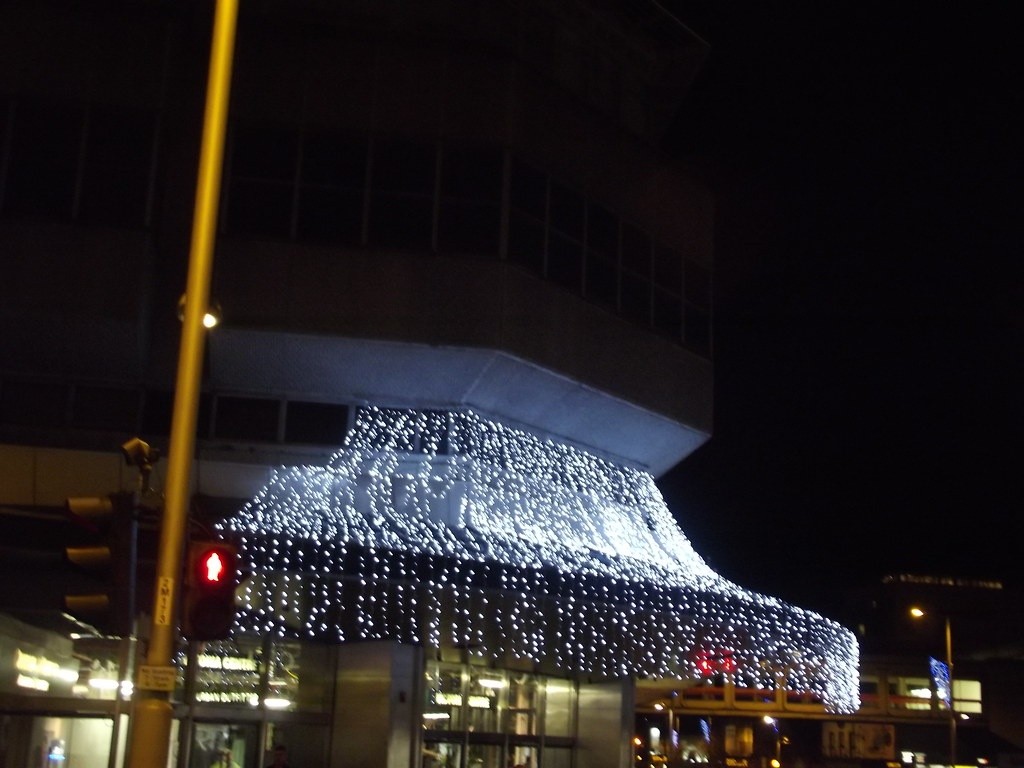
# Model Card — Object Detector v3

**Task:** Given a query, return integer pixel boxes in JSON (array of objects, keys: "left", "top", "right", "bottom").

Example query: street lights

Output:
[{"left": 911, "top": 603, "right": 960, "bottom": 761}]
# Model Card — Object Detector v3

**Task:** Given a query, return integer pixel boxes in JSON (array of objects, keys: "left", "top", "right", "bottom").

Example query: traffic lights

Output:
[
  {"left": 54, "top": 486, "right": 141, "bottom": 633},
  {"left": 178, "top": 542, "right": 246, "bottom": 638}
]
